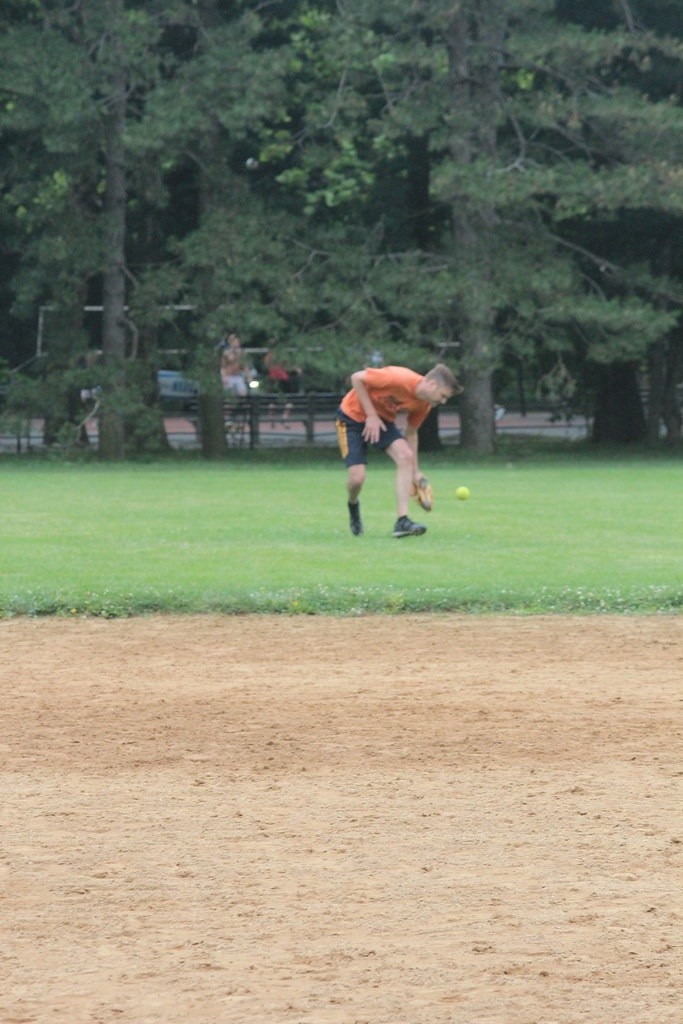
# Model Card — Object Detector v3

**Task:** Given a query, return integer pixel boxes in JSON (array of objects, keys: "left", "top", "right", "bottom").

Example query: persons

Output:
[
  {"left": 335, "top": 362, "right": 463, "bottom": 537},
  {"left": 220, "top": 330, "right": 305, "bottom": 398}
]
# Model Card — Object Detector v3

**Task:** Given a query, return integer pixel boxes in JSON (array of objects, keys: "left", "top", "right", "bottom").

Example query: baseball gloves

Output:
[{"left": 409, "top": 475, "right": 433, "bottom": 512}]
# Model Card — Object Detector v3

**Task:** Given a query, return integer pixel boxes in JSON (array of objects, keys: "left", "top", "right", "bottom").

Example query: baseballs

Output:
[{"left": 455, "top": 486, "right": 470, "bottom": 502}]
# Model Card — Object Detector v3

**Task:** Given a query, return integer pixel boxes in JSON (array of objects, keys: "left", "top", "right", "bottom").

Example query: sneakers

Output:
[
  {"left": 348, "top": 501, "right": 365, "bottom": 536},
  {"left": 392, "top": 516, "right": 426, "bottom": 538}
]
